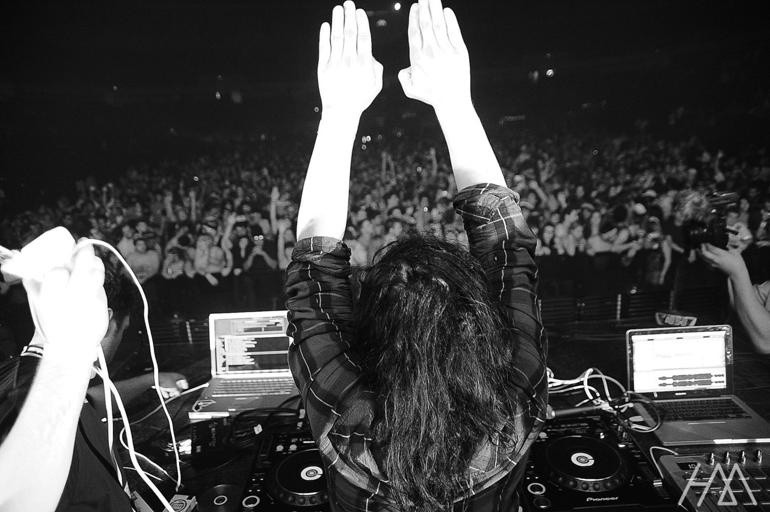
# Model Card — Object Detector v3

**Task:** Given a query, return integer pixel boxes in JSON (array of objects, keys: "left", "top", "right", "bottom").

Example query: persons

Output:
[
  {"left": 0, "top": 235, "right": 108, "bottom": 512},
  {"left": 699, "top": 241, "right": 770, "bottom": 358},
  {"left": 0, "top": 247, "right": 188, "bottom": 512},
  {"left": 1, "top": 132, "right": 769, "bottom": 303},
  {"left": 280, "top": 2, "right": 550, "bottom": 512}
]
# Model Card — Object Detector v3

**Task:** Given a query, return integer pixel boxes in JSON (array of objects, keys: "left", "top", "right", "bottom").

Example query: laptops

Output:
[
  {"left": 191, "top": 311, "right": 302, "bottom": 417},
  {"left": 626, "top": 325, "right": 770, "bottom": 447}
]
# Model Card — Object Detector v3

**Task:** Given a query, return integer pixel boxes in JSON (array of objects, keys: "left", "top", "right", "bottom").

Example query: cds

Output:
[{"left": 198, "top": 484, "right": 244, "bottom": 512}]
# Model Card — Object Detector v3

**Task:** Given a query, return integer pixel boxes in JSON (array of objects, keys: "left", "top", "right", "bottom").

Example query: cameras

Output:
[{"left": 691, "top": 191, "right": 741, "bottom": 250}]
